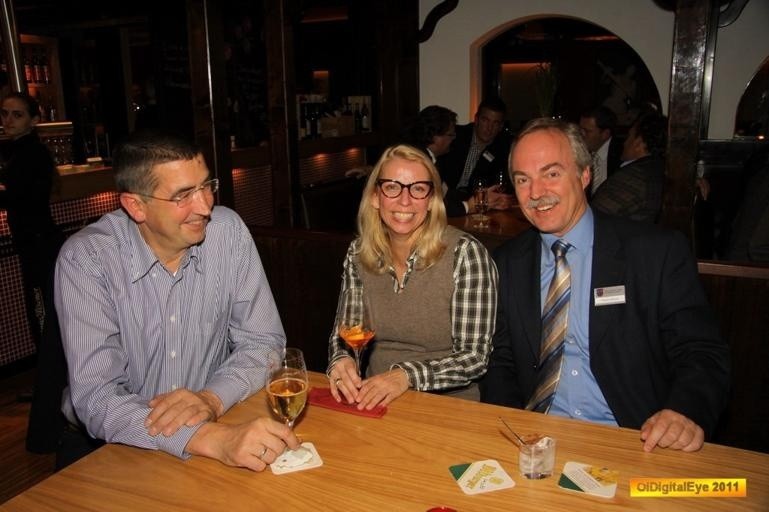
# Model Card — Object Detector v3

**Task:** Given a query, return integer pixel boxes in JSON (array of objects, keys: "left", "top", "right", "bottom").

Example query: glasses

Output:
[
  {"left": 375, "top": 177, "right": 433, "bottom": 200},
  {"left": 132, "top": 177, "right": 220, "bottom": 208}
]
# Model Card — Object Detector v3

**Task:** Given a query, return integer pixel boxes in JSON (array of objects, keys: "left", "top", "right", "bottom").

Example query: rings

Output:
[
  {"left": 259, "top": 446, "right": 268, "bottom": 459},
  {"left": 336, "top": 377, "right": 343, "bottom": 384}
]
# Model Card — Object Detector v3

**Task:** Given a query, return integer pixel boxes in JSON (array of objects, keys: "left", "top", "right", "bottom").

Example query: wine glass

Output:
[
  {"left": 266, "top": 349, "right": 314, "bottom": 470},
  {"left": 471, "top": 169, "right": 508, "bottom": 231},
  {"left": 333, "top": 289, "right": 376, "bottom": 407}
]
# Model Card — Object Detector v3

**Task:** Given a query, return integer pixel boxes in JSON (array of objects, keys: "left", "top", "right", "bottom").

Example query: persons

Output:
[
  {"left": 578, "top": 104, "right": 711, "bottom": 202},
  {"left": 590, "top": 111, "right": 669, "bottom": 223},
  {"left": 395, "top": 105, "right": 514, "bottom": 217},
  {"left": 496, "top": 115, "right": 734, "bottom": 455},
  {"left": 434, "top": 95, "right": 508, "bottom": 200},
  {"left": 723, "top": 164, "right": 769, "bottom": 263},
  {"left": 0, "top": 92, "right": 68, "bottom": 402},
  {"left": 59, "top": 121, "right": 304, "bottom": 473},
  {"left": 324, "top": 142, "right": 501, "bottom": 411}
]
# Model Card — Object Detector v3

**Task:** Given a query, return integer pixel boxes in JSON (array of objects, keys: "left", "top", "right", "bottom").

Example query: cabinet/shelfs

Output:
[{"left": 0, "top": 33, "right": 74, "bottom": 140}]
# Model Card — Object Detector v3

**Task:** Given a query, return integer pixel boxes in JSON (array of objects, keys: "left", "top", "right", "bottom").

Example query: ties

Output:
[
  {"left": 525, "top": 240, "right": 571, "bottom": 415},
  {"left": 592, "top": 151, "right": 600, "bottom": 198}
]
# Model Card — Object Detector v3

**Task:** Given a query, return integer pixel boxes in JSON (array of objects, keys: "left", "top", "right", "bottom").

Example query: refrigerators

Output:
[{"left": 695, "top": 137, "right": 767, "bottom": 261}]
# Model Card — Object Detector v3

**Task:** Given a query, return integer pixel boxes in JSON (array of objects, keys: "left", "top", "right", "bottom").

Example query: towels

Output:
[{"left": 308, "top": 388, "right": 387, "bottom": 418}]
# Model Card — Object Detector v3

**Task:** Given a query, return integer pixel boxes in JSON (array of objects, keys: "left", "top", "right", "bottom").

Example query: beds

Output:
[{"left": 0, "top": 366, "right": 769, "bottom": 512}]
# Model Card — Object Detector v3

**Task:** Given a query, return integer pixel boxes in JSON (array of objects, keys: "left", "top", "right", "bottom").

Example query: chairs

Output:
[
  {"left": 26, "top": 272, "right": 85, "bottom": 471},
  {"left": 298, "top": 176, "right": 367, "bottom": 231},
  {"left": 691, "top": 193, "right": 717, "bottom": 260}
]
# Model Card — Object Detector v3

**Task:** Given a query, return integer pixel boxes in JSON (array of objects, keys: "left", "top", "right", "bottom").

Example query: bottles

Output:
[
  {"left": 303, "top": 100, "right": 372, "bottom": 140},
  {"left": 22, "top": 60, "right": 80, "bottom": 169}
]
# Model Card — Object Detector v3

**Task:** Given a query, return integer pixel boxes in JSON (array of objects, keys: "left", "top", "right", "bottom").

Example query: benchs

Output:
[{"left": 248, "top": 223, "right": 769, "bottom": 453}]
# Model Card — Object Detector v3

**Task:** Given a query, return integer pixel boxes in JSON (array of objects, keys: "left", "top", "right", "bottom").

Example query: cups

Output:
[{"left": 517, "top": 435, "right": 555, "bottom": 480}]
